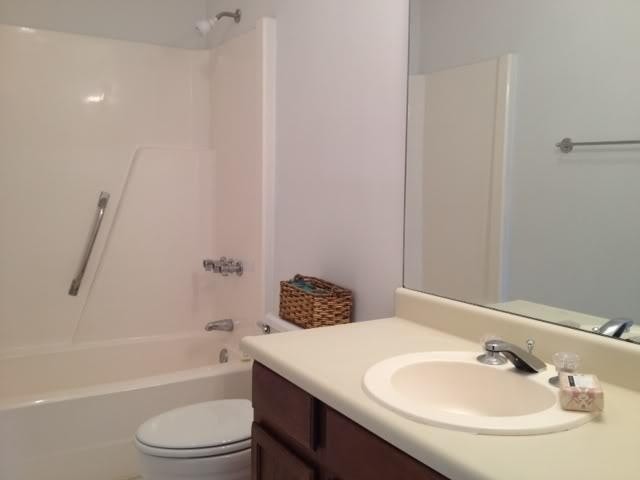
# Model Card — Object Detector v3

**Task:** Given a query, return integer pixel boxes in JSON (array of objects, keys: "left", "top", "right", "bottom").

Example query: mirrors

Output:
[{"left": 401, "top": 1, "right": 638, "bottom": 340}]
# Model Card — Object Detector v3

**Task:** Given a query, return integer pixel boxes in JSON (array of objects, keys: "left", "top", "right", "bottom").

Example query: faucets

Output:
[
  {"left": 593, "top": 317, "right": 639, "bottom": 336},
  {"left": 204, "top": 319, "right": 233, "bottom": 334},
  {"left": 475, "top": 338, "right": 547, "bottom": 374}
]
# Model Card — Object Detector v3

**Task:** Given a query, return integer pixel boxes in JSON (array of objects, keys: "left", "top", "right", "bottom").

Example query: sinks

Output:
[{"left": 361, "top": 349, "right": 602, "bottom": 433}]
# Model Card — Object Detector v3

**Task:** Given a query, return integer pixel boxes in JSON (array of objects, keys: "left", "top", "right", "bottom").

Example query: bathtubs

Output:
[{"left": 1, "top": 319, "right": 274, "bottom": 476}]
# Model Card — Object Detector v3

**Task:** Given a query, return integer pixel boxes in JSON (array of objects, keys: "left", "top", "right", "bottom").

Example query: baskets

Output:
[{"left": 278, "top": 276, "right": 353, "bottom": 329}]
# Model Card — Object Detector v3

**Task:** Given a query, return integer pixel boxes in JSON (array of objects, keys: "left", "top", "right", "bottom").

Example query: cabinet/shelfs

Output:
[{"left": 250, "top": 362, "right": 447, "bottom": 480}]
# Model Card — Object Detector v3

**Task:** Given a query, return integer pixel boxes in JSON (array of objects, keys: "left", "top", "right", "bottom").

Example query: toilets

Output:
[{"left": 134, "top": 394, "right": 252, "bottom": 475}]
